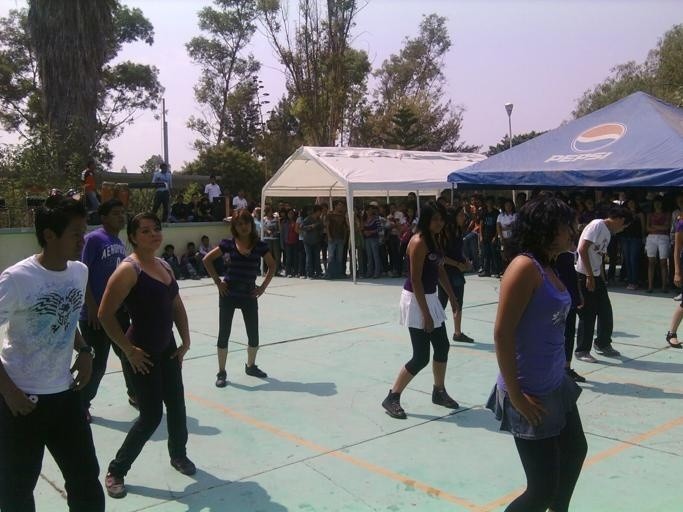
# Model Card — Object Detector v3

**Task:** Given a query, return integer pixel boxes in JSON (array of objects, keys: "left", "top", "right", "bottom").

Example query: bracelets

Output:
[
  {"left": 125, "top": 345, "right": 134, "bottom": 356},
  {"left": 512, "top": 392, "right": 524, "bottom": 405},
  {"left": 181, "top": 344, "right": 189, "bottom": 350},
  {"left": 79, "top": 345, "right": 95, "bottom": 358}
]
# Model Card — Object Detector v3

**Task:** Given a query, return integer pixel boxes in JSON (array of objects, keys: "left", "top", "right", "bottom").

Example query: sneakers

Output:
[
  {"left": 105, "top": 471, "right": 127, "bottom": 498},
  {"left": 245, "top": 362, "right": 267, "bottom": 378},
  {"left": 593, "top": 343, "right": 620, "bottom": 356},
  {"left": 566, "top": 369, "right": 586, "bottom": 381},
  {"left": 577, "top": 353, "right": 597, "bottom": 362},
  {"left": 170, "top": 456, "right": 196, "bottom": 476},
  {"left": 216, "top": 370, "right": 227, "bottom": 387},
  {"left": 453, "top": 333, "right": 474, "bottom": 343}
]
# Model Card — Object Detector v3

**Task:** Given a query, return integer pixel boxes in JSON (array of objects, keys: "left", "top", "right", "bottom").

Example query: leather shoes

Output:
[{"left": 666, "top": 331, "right": 681, "bottom": 348}]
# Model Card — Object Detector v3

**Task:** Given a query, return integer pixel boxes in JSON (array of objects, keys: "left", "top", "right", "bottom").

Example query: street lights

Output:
[
  {"left": 505, "top": 102, "right": 516, "bottom": 148},
  {"left": 153, "top": 97, "right": 171, "bottom": 170}
]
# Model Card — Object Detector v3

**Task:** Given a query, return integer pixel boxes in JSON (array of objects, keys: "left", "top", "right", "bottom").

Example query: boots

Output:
[
  {"left": 432, "top": 384, "right": 458, "bottom": 409},
  {"left": 382, "top": 389, "right": 406, "bottom": 419}
]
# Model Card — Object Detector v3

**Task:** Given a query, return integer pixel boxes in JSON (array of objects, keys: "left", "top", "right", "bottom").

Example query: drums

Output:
[
  {"left": 46, "top": 189, "right": 64, "bottom": 208},
  {"left": 67, "top": 189, "right": 80, "bottom": 201}
]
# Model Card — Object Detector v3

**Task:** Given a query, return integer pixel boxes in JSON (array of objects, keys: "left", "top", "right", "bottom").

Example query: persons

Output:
[
  {"left": 437, "top": 189, "right": 474, "bottom": 343},
  {"left": 666, "top": 219, "right": 683, "bottom": 348},
  {"left": 51, "top": 188, "right": 76, "bottom": 197},
  {"left": 553, "top": 190, "right": 683, "bottom": 300},
  {"left": 161, "top": 235, "right": 230, "bottom": 280},
  {"left": 461, "top": 191, "right": 548, "bottom": 278},
  {"left": 171, "top": 177, "right": 247, "bottom": 222},
  {"left": 82, "top": 163, "right": 98, "bottom": 219},
  {"left": 98, "top": 211, "right": 195, "bottom": 497},
  {"left": 253, "top": 192, "right": 422, "bottom": 279},
  {"left": 382, "top": 200, "right": 460, "bottom": 419},
  {"left": 203, "top": 208, "right": 276, "bottom": 387},
  {"left": 82, "top": 198, "right": 143, "bottom": 420},
  {"left": 486, "top": 192, "right": 587, "bottom": 511},
  {"left": 152, "top": 164, "right": 172, "bottom": 222},
  {"left": 1, "top": 194, "right": 104, "bottom": 511},
  {"left": 556, "top": 208, "right": 634, "bottom": 381}
]
